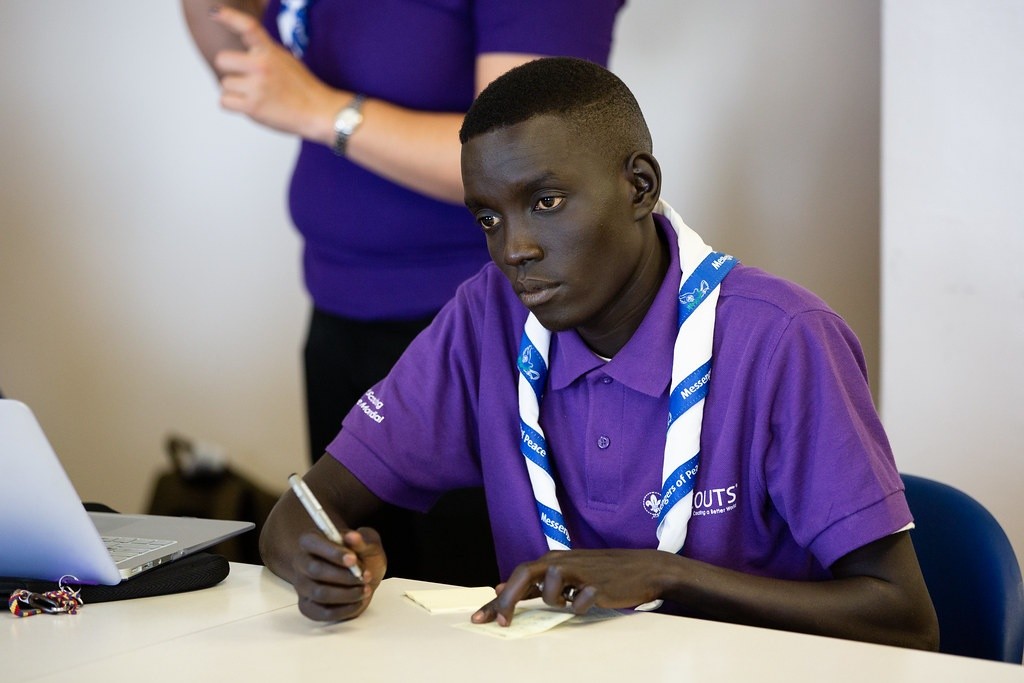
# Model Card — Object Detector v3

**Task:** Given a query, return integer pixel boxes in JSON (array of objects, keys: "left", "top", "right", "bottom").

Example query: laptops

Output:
[{"left": 0, "top": 398, "right": 256, "bottom": 585}]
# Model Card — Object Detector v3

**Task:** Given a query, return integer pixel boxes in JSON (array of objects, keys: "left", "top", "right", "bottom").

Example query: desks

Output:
[{"left": 1, "top": 561, "right": 1023, "bottom": 683}]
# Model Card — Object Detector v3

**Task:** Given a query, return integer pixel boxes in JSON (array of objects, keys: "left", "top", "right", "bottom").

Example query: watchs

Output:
[{"left": 333, "top": 94, "right": 370, "bottom": 158}]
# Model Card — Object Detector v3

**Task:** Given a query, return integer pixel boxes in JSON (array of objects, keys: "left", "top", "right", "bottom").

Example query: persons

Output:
[
  {"left": 176, "top": 0, "right": 631, "bottom": 574},
  {"left": 259, "top": 57, "right": 940, "bottom": 652}
]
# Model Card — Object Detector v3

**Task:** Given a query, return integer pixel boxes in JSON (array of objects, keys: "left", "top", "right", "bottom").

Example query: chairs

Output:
[{"left": 895, "top": 472, "right": 1024, "bottom": 663}]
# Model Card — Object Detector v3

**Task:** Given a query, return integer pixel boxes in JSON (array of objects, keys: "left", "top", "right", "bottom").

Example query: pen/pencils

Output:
[{"left": 287, "top": 472, "right": 364, "bottom": 580}]
